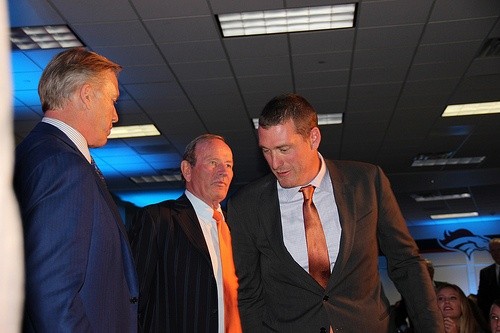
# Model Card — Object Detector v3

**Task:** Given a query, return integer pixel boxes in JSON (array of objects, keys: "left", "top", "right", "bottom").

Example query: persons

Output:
[
  {"left": 135, "top": 134, "right": 244, "bottom": 333},
  {"left": 391, "top": 235, "right": 500, "bottom": 333},
  {"left": 12, "top": 49, "right": 139, "bottom": 333},
  {"left": 228, "top": 94, "right": 445, "bottom": 333}
]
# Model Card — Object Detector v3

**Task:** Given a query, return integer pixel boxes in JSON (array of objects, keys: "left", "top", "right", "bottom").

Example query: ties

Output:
[
  {"left": 213, "top": 208, "right": 242, "bottom": 333},
  {"left": 298, "top": 184, "right": 332, "bottom": 333},
  {"left": 90, "top": 155, "right": 106, "bottom": 189}
]
